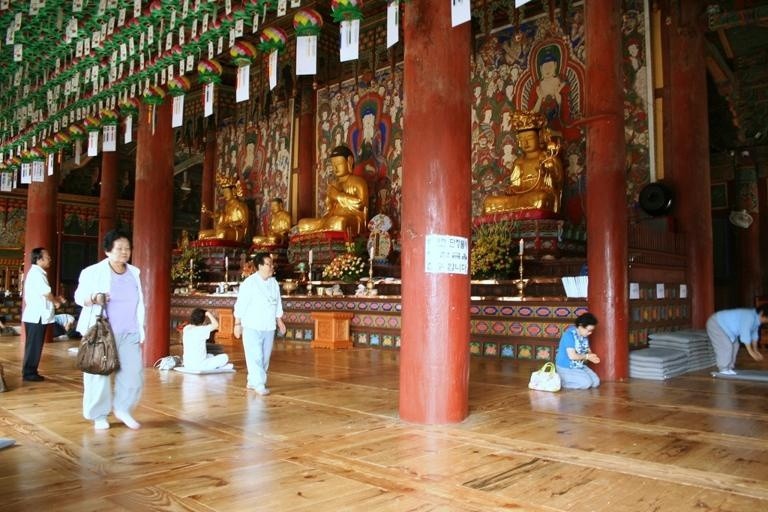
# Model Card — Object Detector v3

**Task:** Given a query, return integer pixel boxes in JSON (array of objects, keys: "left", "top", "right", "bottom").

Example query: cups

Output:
[{"left": 316, "top": 287, "right": 333, "bottom": 296}]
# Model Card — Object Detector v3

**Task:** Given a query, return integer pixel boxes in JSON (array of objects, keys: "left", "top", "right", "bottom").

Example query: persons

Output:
[
  {"left": 234, "top": 139, "right": 267, "bottom": 195},
  {"left": 252, "top": 196, "right": 293, "bottom": 246},
  {"left": 295, "top": 144, "right": 369, "bottom": 236},
  {"left": 197, "top": 184, "right": 250, "bottom": 242},
  {"left": 526, "top": 48, "right": 582, "bottom": 142},
  {"left": 73, "top": 228, "right": 146, "bottom": 431},
  {"left": 705, "top": 302, "right": 768, "bottom": 376},
  {"left": 53, "top": 313, "right": 81, "bottom": 339},
  {"left": 480, "top": 110, "right": 568, "bottom": 213},
  {"left": 232, "top": 251, "right": 287, "bottom": 394},
  {"left": 352, "top": 107, "right": 391, "bottom": 183},
  {"left": 554, "top": 312, "right": 602, "bottom": 390},
  {"left": 20, "top": 247, "right": 68, "bottom": 381},
  {"left": 182, "top": 307, "right": 235, "bottom": 372}
]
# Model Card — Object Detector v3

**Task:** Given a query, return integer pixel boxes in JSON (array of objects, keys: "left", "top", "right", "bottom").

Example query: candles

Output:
[
  {"left": 519, "top": 236, "right": 524, "bottom": 255},
  {"left": 309, "top": 250, "right": 313, "bottom": 264},
  {"left": 225, "top": 256, "right": 229, "bottom": 267},
  {"left": 370, "top": 246, "right": 374, "bottom": 259},
  {"left": 190, "top": 258, "right": 193, "bottom": 268}
]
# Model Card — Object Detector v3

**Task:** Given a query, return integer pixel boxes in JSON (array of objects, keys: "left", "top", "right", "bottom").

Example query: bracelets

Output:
[
  {"left": 234, "top": 322, "right": 242, "bottom": 326},
  {"left": 90, "top": 292, "right": 97, "bottom": 305},
  {"left": 583, "top": 353, "right": 589, "bottom": 361}
]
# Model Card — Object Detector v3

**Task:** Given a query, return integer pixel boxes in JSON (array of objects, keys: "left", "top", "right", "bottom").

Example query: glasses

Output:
[{"left": 583, "top": 327, "right": 594, "bottom": 332}]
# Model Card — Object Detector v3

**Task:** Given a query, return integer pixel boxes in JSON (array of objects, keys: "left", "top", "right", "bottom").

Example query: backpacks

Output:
[{"left": 76, "top": 315, "right": 120, "bottom": 376}]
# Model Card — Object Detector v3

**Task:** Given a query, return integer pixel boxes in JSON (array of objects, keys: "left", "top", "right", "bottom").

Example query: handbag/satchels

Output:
[
  {"left": 528, "top": 362, "right": 561, "bottom": 392},
  {"left": 156, "top": 356, "right": 180, "bottom": 370}
]
